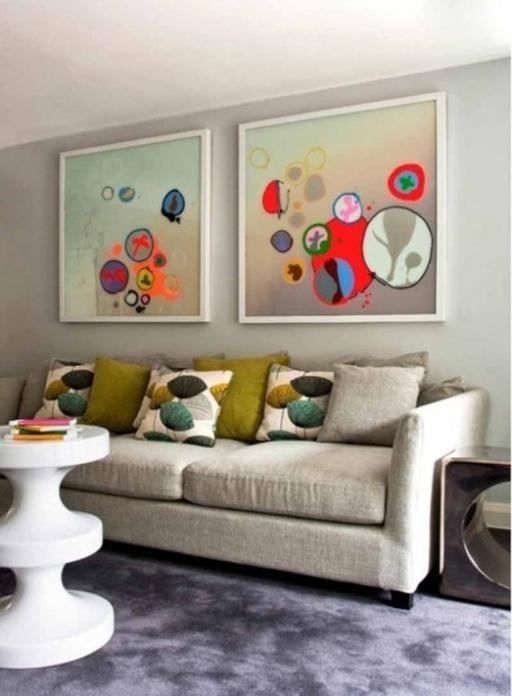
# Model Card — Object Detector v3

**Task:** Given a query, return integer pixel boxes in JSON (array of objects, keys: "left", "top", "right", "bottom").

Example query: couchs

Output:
[{"left": 0, "top": 369, "right": 491, "bottom": 610}]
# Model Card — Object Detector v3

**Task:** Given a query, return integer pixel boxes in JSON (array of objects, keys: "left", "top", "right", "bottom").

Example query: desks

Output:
[
  {"left": 437, "top": 448, "right": 510, "bottom": 608},
  {"left": 1, "top": 426, "right": 114, "bottom": 667}
]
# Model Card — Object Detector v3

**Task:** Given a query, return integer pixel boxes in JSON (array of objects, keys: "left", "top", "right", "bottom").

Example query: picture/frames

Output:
[
  {"left": 58, "top": 128, "right": 210, "bottom": 324},
  {"left": 237, "top": 92, "right": 453, "bottom": 323}
]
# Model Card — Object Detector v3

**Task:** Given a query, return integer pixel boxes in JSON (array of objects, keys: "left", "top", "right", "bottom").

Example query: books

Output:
[{"left": 4, "top": 417, "right": 78, "bottom": 440}]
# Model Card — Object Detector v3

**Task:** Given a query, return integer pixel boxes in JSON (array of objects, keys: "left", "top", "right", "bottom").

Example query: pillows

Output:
[
  {"left": 193, "top": 350, "right": 290, "bottom": 441},
  {"left": 255, "top": 362, "right": 335, "bottom": 440},
  {"left": 135, "top": 369, "right": 235, "bottom": 446},
  {"left": 417, "top": 375, "right": 463, "bottom": 410},
  {"left": 289, "top": 354, "right": 354, "bottom": 372},
  {"left": 81, "top": 352, "right": 149, "bottom": 432},
  {"left": 131, "top": 360, "right": 183, "bottom": 429},
  {"left": 315, "top": 363, "right": 424, "bottom": 446},
  {"left": 31, "top": 356, "right": 95, "bottom": 425},
  {"left": 149, "top": 352, "right": 226, "bottom": 374},
  {"left": 111, "top": 353, "right": 166, "bottom": 369},
  {"left": 19, "top": 368, "right": 49, "bottom": 419},
  {"left": 354, "top": 350, "right": 431, "bottom": 378}
]
